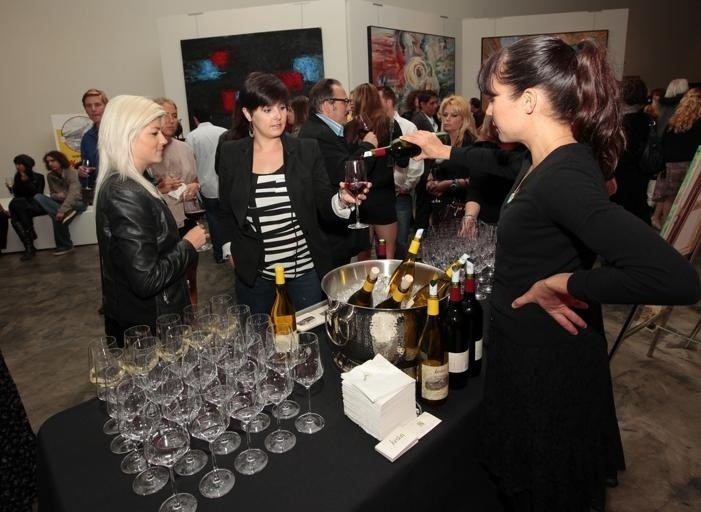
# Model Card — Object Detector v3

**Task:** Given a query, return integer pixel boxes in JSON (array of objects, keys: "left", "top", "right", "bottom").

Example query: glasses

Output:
[{"left": 324, "top": 97, "right": 350, "bottom": 104}]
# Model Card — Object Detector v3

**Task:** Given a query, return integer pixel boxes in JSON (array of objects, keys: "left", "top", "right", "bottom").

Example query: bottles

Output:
[
  {"left": 380, "top": 226, "right": 426, "bottom": 304},
  {"left": 348, "top": 267, "right": 378, "bottom": 309},
  {"left": 373, "top": 272, "right": 412, "bottom": 310},
  {"left": 457, "top": 258, "right": 483, "bottom": 382},
  {"left": 409, "top": 251, "right": 471, "bottom": 311},
  {"left": 439, "top": 268, "right": 471, "bottom": 394},
  {"left": 411, "top": 278, "right": 449, "bottom": 411},
  {"left": 358, "top": 130, "right": 451, "bottom": 158},
  {"left": 269, "top": 265, "right": 301, "bottom": 363}
]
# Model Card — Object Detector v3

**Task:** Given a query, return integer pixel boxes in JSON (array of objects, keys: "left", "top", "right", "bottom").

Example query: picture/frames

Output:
[
  {"left": 480, "top": 30, "right": 609, "bottom": 69},
  {"left": 367, "top": 26, "right": 455, "bottom": 98}
]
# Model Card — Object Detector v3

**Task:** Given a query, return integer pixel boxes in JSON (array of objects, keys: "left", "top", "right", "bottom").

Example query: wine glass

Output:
[
  {"left": 428, "top": 168, "right": 443, "bottom": 204},
  {"left": 416, "top": 216, "right": 497, "bottom": 300},
  {"left": 353, "top": 112, "right": 373, "bottom": 135},
  {"left": 182, "top": 187, "right": 213, "bottom": 253},
  {"left": 343, "top": 159, "right": 369, "bottom": 231},
  {"left": 85, "top": 291, "right": 324, "bottom": 512},
  {"left": 5, "top": 175, "right": 15, "bottom": 197},
  {"left": 78, "top": 160, "right": 94, "bottom": 191}
]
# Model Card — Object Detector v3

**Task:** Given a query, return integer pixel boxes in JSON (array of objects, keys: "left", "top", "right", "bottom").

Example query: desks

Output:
[{"left": 39, "top": 325, "right": 486, "bottom": 512}]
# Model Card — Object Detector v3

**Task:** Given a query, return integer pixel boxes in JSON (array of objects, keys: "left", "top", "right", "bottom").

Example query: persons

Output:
[
  {"left": 152, "top": 97, "right": 229, "bottom": 264},
  {"left": 2, "top": 89, "right": 107, "bottom": 260},
  {"left": 92, "top": 93, "right": 213, "bottom": 353},
  {"left": 399, "top": 34, "right": 701, "bottom": 512},
  {"left": 606, "top": 77, "right": 700, "bottom": 230},
  {"left": 285, "top": 76, "right": 498, "bottom": 263},
  {"left": 218, "top": 70, "right": 373, "bottom": 340}
]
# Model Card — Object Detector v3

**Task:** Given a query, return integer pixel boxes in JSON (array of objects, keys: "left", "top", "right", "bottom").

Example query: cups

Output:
[{"left": 167, "top": 158, "right": 182, "bottom": 181}]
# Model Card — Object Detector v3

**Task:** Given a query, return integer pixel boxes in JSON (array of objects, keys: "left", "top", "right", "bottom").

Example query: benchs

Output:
[{"left": 2, "top": 206, "right": 98, "bottom": 253}]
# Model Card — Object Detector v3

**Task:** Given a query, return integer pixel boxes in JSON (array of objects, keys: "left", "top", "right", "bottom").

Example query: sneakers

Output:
[
  {"left": 60, "top": 209, "right": 78, "bottom": 225},
  {"left": 21, "top": 231, "right": 38, "bottom": 242},
  {"left": 214, "top": 253, "right": 227, "bottom": 264},
  {"left": 52, "top": 242, "right": 74, "bottom": 257}
]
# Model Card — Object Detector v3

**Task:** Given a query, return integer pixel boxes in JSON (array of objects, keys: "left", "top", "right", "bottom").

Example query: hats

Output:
[{"left": 13, "top": 154, "right": 35, "bottom": 167}]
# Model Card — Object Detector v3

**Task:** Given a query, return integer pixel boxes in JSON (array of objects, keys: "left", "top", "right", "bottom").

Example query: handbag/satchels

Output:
[{"left": 229, "top": 234, "right": 266, "bottom": 270}]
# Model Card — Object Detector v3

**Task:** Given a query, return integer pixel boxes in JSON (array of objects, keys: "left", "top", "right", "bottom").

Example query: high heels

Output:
[{"left": 21, "top": 248, "right": 36, "bottom": 260}]
